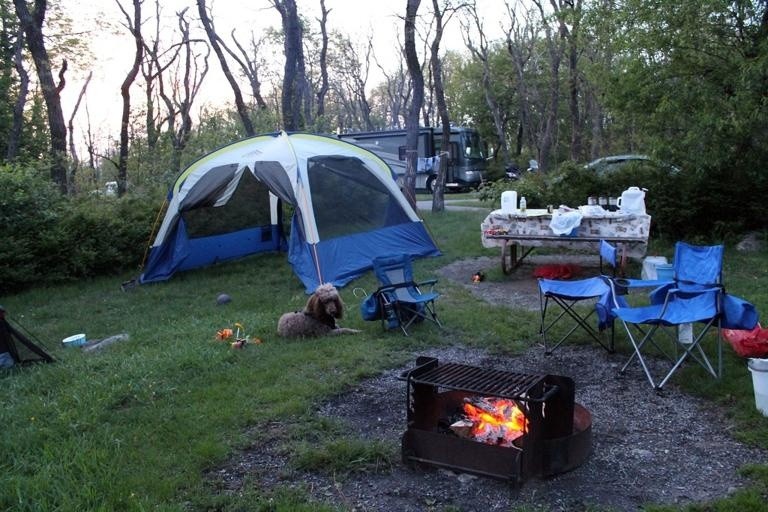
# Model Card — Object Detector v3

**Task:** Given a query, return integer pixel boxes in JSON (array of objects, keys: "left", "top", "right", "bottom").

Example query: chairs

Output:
[
  {"left": 608, "top": 239, "right": 726, "bottom": 395},
  {"left": 371, "top": 252, "right": 447, "bottom": 338},
  {"left": 530, "top": 236, "right": 623, "bottom": 361}
]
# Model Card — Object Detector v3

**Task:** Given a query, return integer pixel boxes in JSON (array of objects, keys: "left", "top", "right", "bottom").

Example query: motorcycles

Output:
[{"left": 503, "top": 159, "right": 539, "bottom": 182}]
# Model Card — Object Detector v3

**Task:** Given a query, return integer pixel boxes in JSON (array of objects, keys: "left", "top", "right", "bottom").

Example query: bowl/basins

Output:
[{"left": 62, "top": 332, "right": 86, "bottom": 348}]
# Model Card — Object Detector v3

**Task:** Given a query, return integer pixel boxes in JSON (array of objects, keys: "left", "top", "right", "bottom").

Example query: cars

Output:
[{"left": 554, "top": 156, "right": 681, "bottom": 181}]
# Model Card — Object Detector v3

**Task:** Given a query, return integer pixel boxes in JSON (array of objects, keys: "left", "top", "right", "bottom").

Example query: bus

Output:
[{"left": 334, "top": 126, "right": 492, "bottom": 193}]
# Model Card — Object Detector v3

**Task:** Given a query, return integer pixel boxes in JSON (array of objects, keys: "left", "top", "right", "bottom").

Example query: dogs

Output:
[{"left": 277, "top": 282, "right": 362, "bottom": 339}]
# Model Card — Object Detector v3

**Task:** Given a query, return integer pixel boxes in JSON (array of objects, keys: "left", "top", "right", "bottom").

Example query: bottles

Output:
[{"left": 520, "top": 196, "right": 526, "bottom": 214}]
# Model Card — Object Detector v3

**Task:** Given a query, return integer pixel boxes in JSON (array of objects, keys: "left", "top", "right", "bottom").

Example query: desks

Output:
[{"left": 485, "top": 206, "right": 652, "bottom": 281}]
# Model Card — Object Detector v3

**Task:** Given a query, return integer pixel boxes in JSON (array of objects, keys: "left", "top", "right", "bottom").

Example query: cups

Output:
[
  {"left": 587, "top": 196, "right": 616, "bottom": 212},
  {"left": 546, "top": 205, "right": 552, "bottom": 214}
]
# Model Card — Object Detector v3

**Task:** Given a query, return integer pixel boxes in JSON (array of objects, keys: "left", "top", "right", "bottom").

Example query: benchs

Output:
[{"left": 483, "top": 232, "right": 646, "bottom": 280}]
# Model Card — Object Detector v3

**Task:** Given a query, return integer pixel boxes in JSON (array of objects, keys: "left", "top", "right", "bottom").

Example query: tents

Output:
[{"left": 121, "top": 129, "right": 443, "bottom": 297}]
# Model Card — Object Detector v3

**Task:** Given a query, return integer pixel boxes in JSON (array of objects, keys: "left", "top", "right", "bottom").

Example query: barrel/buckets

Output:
[
  {"left": 500, "top": 190, "right": 518, "bottom": 212},
  {"left": 655, "top": 264, "right": 675, "bottom": 281},
  {"left": 746, "top": 357, "right": 768, "bottom": 419}
]
